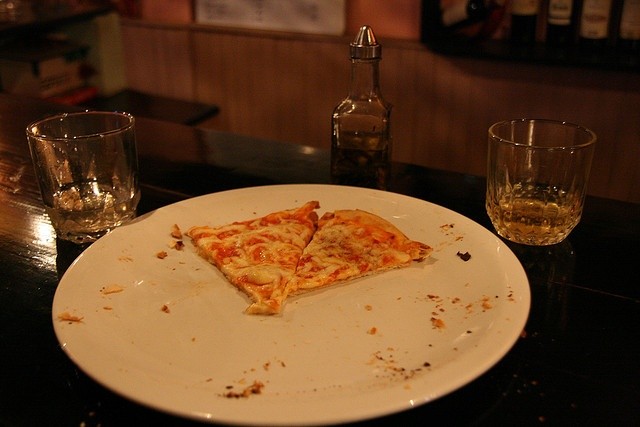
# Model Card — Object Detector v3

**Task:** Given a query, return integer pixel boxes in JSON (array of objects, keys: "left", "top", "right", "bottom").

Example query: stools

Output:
[{"left": 89, "top": 89, "right": 220, "bottom": 126}]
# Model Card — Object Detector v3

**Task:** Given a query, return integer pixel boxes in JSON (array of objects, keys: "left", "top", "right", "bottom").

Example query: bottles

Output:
[{"left": 331, "top": 26, "right": 394, "bottom": 192}]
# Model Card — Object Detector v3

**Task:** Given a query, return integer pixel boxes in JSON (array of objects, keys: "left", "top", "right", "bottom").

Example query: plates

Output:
[{"left": 51, "top": 182, "right": 531, "bottom": 427}]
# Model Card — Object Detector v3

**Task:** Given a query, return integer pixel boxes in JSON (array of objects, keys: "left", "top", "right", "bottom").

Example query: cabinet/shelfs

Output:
[
  {"left": 1, "top": 0, "right": 126, "bottom": 104},
  {"left": 419, "top": 0, "right": 639, "bottom": 73}
]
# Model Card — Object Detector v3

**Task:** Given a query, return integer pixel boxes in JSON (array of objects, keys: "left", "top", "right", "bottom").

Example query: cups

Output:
[
  {"left": 26, "top": 112, "right": 142, "bottom": 245},
  {"left": 485, "top": 118, "right": 599, "bottom": 245}
]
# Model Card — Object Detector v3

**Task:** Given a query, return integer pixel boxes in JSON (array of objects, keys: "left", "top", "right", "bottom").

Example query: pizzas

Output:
[
  {"left": 185, "top": 199, "right": 322, "bottom": 315},
  {"left": 286, "top": 208, "right": 433, "bottom": 296}
]
